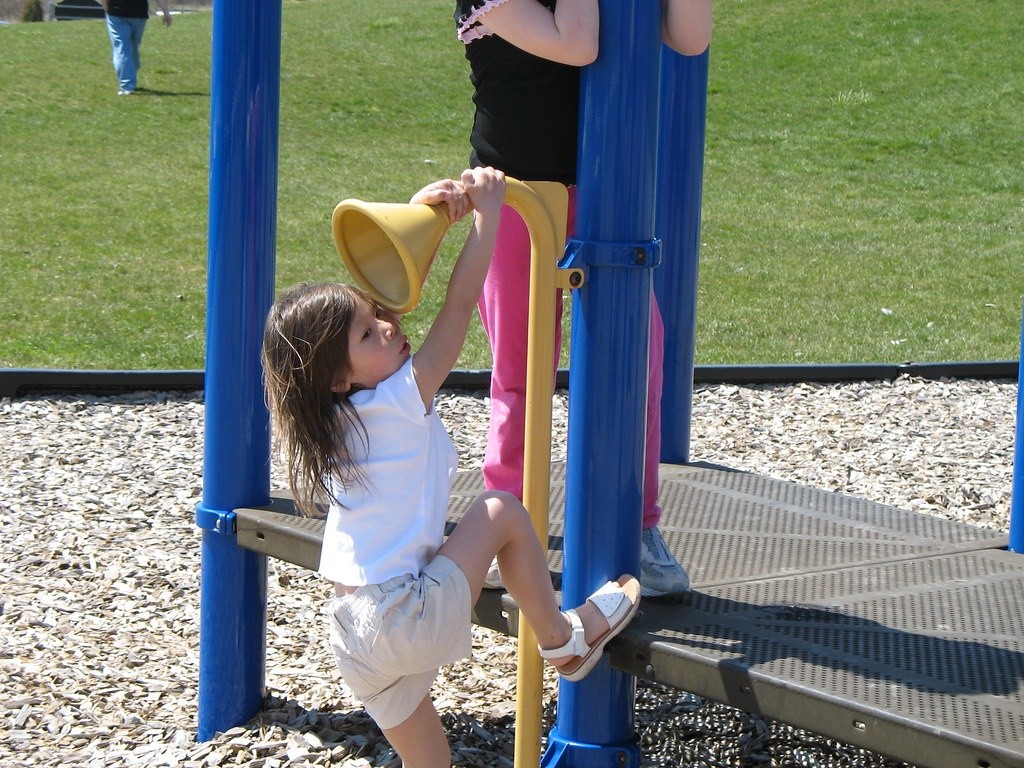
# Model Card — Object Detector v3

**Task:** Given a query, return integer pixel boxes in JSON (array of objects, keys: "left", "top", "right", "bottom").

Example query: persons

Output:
[
  {"left": 96, "top": 0, "right": 172, "bottom": 95},
  {"left": 453, "top": 1, "right": 713, "bottom": 597},
  {"left": 260, "top": 166, "right": 641, "bottom": 768}
]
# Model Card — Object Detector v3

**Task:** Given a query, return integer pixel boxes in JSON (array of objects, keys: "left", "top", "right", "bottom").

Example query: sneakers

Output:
[
  {"left": 641, "top": 526, "right": 691, "bottom": 596},
  {"left": 482, "top": 555, "right": 506, "bottom": 589}
]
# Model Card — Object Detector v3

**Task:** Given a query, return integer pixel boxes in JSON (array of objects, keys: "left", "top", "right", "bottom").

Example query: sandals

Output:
[{"left": 538, "top": 573, "right": 641, "bottom": 682}]
